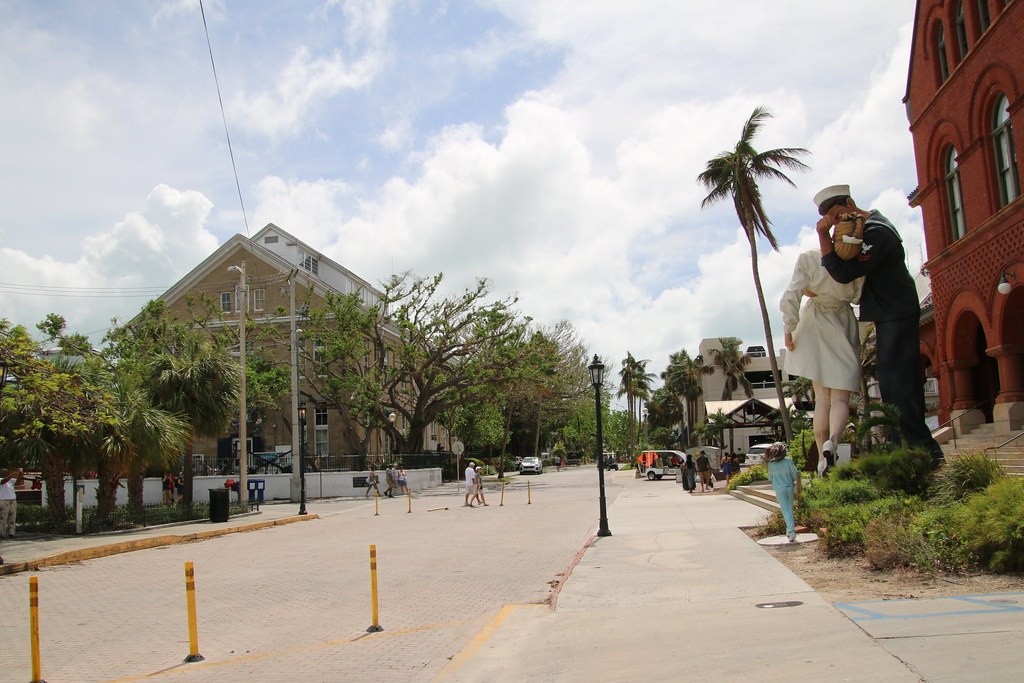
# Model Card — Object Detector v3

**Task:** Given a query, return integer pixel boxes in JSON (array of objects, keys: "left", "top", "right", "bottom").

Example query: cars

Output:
[
  {"left": 744, "top": 443, "right": 773, "bottom": 466},
  {"left": 519, "top": 456, "right": 543, "bottom": 475},
  {"left": 565, "top": 451, "right": 583, "bottom": 466}
]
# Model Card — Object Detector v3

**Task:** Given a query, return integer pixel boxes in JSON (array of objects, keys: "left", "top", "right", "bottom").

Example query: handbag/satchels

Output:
[
  {"left": 694, "top": 475, "right": 700, "bottom": 482},
  {"left": 711, "top": 473, "right": 716, "bottom": 483}
]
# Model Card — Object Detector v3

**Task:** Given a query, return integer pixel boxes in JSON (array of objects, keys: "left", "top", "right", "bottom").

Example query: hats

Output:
[{"left": 476, "top": 467, "right": 481, "bottom": 471}]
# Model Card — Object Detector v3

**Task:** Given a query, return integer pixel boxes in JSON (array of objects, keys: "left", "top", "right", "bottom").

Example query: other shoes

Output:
[
  {"left": 690, "top": 489, "right": 692, "bottom": 492},
  {"left": 389, "top": 495, "right": 394, "bottom": 498},
  {"left": 788, "top": 532, "right": 795, "bottom": 542},
  {"left": 384, "top": 492, "right": 387, "bottom": 495},
  {"left": 8, "top": 534, "right": 16, "bottom": 538}
]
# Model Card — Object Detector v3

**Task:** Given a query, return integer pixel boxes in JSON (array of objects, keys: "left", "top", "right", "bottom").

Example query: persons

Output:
[
  {"left": 365, "top": 466, "right": 383, "bottom": 497},
  {"left": 365, "top": 453, "right": 401, "bottom": 471},
  {"left": 608, "top": 456, "right": 615, "bottom": 464},
  {"left": 816, "top": 185, "right": 946, "bottom": 472},
  {"left": 650, "top": 455, "right": 658, "bottom": 468},
  {"left": 682, "top": 454, "right": 697, "bottom": 493},
  {"left": 437, "top": 443, "right": 445, "bottom": 453},
  {"left": 384, "top": 464, "right": 408, "bottom": 498},
  {"left": 0, "top": 468, "right": 24, "bottom": 539},
  {"left": 696, "top": 451, "right": 712, "bottom": 493},
  {"left": 465, "top": 462, "right": 489, "bottom": 507},
  {"left": 161, "top": 472, "right": 184, "bottom": 506},
  {"left": 315, "top": 454, "right": 321, "bottom": 472},
  {"left": 731, "top": 452, "right": 740, "bottom": 478},
  {"left": 779, "top": 211, "right": 865, "bottom": 481},
  {"left": 671, "top": 455, "right": 680, "bottom": 467},
  {"left": 721, "top": 451, "right": 731, "bottom": 483},
  {"left": 768, "top": 442, "right": 801, "bottom": 542},
  {"left": 233, "top": 444, "right": 237, "bottom": 458},
  {"left": 556, "top": 455, "right": 561, "bottom": 472},
  {"left": 657, "top": 456, "right": 663, "bottom": 467}
]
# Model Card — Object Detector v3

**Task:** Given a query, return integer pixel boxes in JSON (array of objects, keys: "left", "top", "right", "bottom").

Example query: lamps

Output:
[{"left": 997, "top": 270, "right": 1016, "bottom": 295}]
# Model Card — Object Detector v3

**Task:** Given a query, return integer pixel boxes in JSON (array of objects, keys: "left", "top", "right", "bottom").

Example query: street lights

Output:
[
  {"left": 588, "top": 353, "right": 612, "bottom": 536},
  {"left": 297, "top": 402, "right": 308, "bottom": 514},
  {"left": 228, "top": 260, "right": 248, "bottom": 513},
  {"left": 389, "top": 411, "right": 397, "bottom": 468}
]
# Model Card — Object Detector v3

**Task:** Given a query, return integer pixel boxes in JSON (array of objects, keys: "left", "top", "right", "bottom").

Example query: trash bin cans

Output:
[
  {"left": 15, "top": 489, "right": 42, "bottom": 531},
  {"left": 208, "top": 488, "right": 230, "bottom": 523}
]
